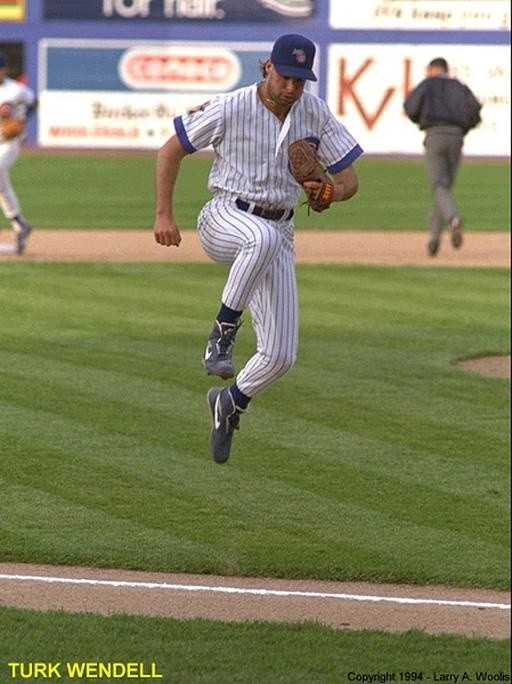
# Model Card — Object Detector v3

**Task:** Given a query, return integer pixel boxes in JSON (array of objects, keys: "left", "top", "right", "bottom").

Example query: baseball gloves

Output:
[
  {"left": 288, "top": 139, "right": 332, "bottom": 212},
  {"left": 1, "top": 118, "right": 24, "bottom": 139}
]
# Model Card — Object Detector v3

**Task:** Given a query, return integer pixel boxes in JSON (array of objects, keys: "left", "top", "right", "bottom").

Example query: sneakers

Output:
[
  {"left": 450, "top": 216, "right": 462, "bottom": 248},
  {"left": 17, "top": 221, "right": 32, "bottom": 253},
  {"left": 427, "top": 239, "right": 438, "bottom": 254},
  {"left": 206, "top": 385, "right": 246, "bottom": 463},
  {"left": 200, "top": 316, "right": 245, "bottom": 380}
]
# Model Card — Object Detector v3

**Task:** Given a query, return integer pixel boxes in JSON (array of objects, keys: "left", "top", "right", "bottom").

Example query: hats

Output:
[{"left": 270, "top": 33, "right": 318, "bottom": 81}]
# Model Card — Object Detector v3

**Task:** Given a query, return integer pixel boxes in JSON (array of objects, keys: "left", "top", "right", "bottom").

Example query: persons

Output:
[
  {"left": 0, "top": 49, "right": 39, "bottom": 255},
  {"left": 153, "top": 35, "right": 364, "bottom": 463},
  {"left": 402, "top": 58, "right": 483, "bottom": 255}
]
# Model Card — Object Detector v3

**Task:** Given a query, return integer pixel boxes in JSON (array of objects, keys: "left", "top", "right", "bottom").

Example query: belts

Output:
[{"left": 235, "top": 197, "right": 293, "bottom": 220}]
